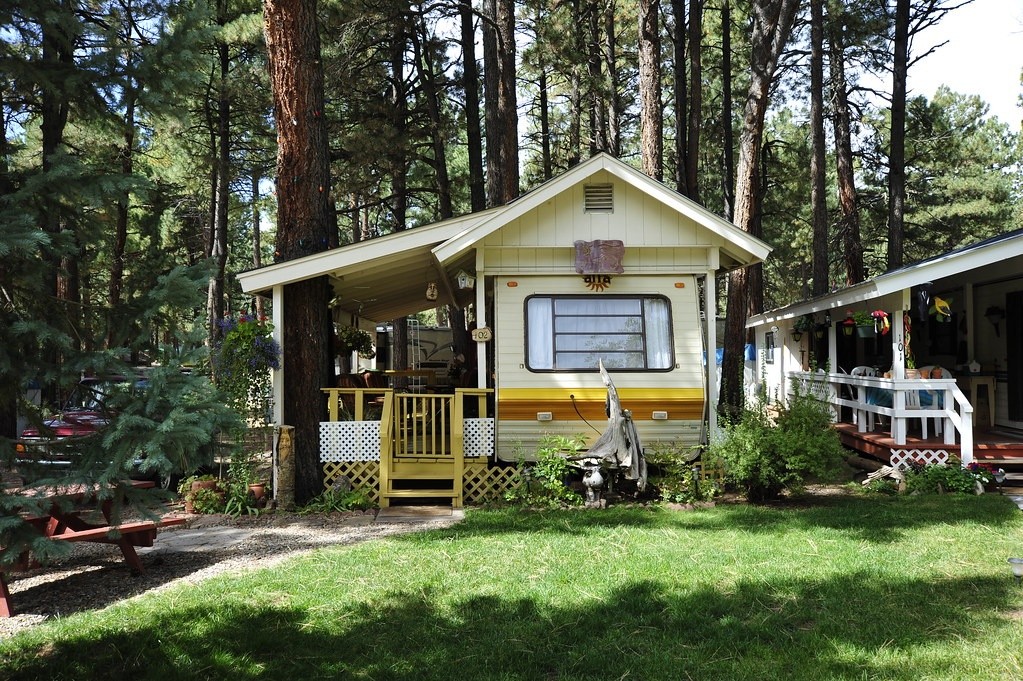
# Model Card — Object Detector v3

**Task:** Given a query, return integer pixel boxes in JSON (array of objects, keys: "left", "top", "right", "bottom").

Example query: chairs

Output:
[
  {"left": 839, "top": 366, "right": 867, "bottom": 425},
  {"left": 919, "top": 366, "right": 952, "bottom": 433},
  {"left": 851, "top": 366, "right": 885, "bottom": 427}
]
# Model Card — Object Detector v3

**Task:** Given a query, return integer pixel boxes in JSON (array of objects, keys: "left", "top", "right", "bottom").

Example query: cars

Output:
[{"left": 13, "top": 373, "right": 149, "bottom": 487}]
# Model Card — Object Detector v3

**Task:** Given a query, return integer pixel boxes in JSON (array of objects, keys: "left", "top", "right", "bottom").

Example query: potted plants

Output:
[
  {"left": 188, "top": 474, "right": 216, "bottom": 493},
  {"left": 212, "top": 491, "right": 227, "bottom": 508},
  {"left": 185, "top": 494, "right": 199, "bottom": 513},
  {"left": 853, "top": 310, "right": 875, "bottom": 337},
  {"left": 248, "top": 476, "right": 265, "bottom": 506}
]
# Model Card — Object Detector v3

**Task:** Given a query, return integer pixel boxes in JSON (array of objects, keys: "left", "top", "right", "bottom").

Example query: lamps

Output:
[
  {"left": 813, "top": 325, "right": 823, "bottom": 341},
  {"left": 843, "top": 318, "right": 853, "bottom": 336},
  {"left": 791, "top": 329, "right": 802, "bottom": 342},
  {"left": 824, "top": 310, "right": 831, "bottom": 326}
]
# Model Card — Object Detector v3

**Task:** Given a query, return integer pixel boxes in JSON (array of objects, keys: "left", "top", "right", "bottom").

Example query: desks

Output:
[{"left": 954, "top": 370, "right": 996, "bottom": 427}]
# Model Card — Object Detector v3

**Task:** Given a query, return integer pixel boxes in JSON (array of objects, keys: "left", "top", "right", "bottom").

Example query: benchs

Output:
[{"left": 0, "top": 480, "right": 186, "bottom": 617}]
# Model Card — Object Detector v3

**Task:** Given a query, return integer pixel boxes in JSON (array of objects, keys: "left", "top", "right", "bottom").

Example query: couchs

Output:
[
  {"left": 364, "top": 371, "right": 414, "bottom": 411},
  {"left": 336, "top": 374, "right": 383, "bottom": 420}
]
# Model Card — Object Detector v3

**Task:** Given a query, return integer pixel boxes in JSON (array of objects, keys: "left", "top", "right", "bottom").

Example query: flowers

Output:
[{"left": 211, "top": 310, "right": 281, "bottom": 380}]
